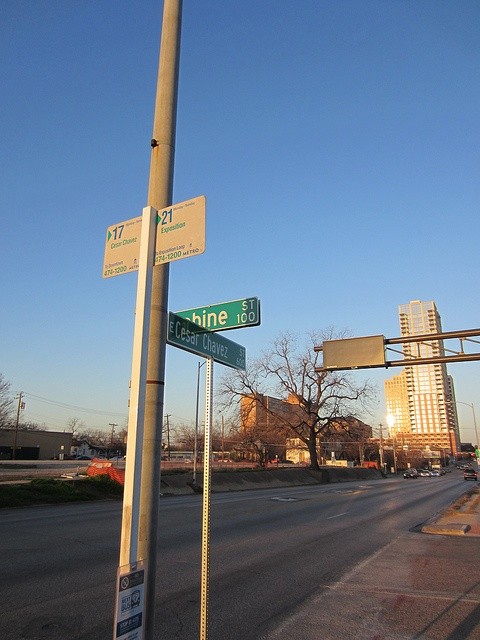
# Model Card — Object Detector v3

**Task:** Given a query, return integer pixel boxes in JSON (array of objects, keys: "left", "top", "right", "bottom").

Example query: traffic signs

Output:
[
  {"left": 166, "top": 312, "right": 246, "bottom": 371},
  {"left": 172, "top": 296, "right": 261, "bottom": 332}
]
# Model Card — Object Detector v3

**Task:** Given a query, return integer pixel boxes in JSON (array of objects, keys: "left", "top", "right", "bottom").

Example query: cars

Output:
[
  {"left": 440, "top": 469, "right": 451, "bottom": 476},
  {"left": 420, "top": 470, "right": 430, "bottom": 477},
  {"left": 403, "top": 468, "right": 418, "bottom": 479},
  {"left": 463, "top": 469, "right": 478, "bottom": 481},
  {"left": 430, "top": 470, "right": 440, "bottom": 477},
  {"left": 456, "top": 461, "right": 472, "bottom": 470}
]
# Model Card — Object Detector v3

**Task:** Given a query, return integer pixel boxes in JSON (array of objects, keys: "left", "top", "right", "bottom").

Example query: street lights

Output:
[{"left": 448, "top": 400, "right": 480, "bottom": 460}]
